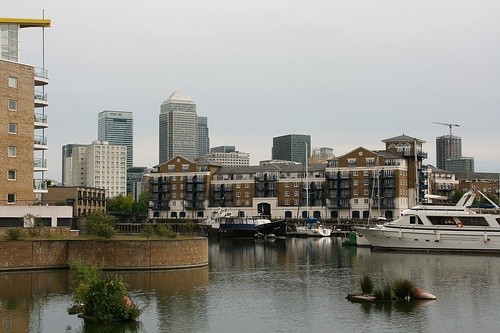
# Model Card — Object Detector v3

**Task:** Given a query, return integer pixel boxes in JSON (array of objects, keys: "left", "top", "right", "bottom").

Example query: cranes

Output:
[{"left": 431, "top": 121, "right": 460, "bottom": 136}]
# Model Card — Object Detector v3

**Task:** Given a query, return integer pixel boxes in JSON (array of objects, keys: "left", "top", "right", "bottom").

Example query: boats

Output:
[
  {"left": 212, "top": 216, "right": 272, "bottom": 235},
  {"left": 252, "top": 219, "right": 287, "bottom": 240},
  {"left": 349, "top": 186, "right": 499, "bottom": 254}
]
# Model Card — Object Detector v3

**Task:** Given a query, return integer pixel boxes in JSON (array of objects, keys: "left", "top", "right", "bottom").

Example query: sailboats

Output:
[
  {"left": 283, "top": 143, "right": 348, "bottom": 238},
  {"left": 349, "top": 155, "right": 388, "bottom": 247}
]
